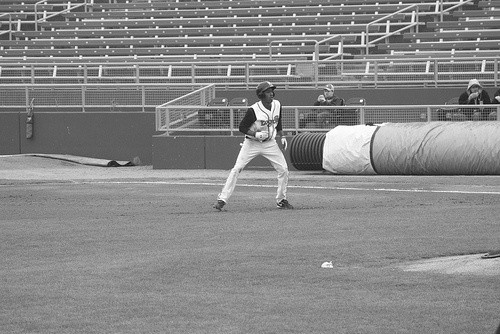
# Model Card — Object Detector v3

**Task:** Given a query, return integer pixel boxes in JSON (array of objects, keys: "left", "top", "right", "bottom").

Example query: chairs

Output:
[
  {"left": 324, "top": 97, "right": 367, "bottom": 125},
  {"left": 437, "top": 97, "right": 492, "bottom": 120},
  {"left": 199, "top": 97, "right": 248, "bottom": 127}
]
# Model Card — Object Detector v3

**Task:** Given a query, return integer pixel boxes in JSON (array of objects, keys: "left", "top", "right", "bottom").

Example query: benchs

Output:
[{"left": 0, "top": 0, "right": 500, "bottom": 85}]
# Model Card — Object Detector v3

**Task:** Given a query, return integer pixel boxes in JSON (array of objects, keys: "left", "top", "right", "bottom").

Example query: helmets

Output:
[{"left": 256, "top": 81, "right": 276, "bottom": 100}]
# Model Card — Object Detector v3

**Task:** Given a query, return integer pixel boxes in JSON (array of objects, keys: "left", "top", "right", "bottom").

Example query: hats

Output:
[{"left": 324, "top": 83, "right": 335, "bottom": 92}]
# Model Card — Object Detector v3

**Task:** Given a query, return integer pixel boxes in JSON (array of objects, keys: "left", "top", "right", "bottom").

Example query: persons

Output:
[
  {"left": 213, "top": 82, "right": 294, "bottom": 211},
  {"left": 292, "top": 84, "right": 341, "bottom": 135},
  {"left": 492, "top": 88, "right": 500, "bottom": 104},
  {"left": 459, "top": 79, "right": 496, "bottom": 121}
]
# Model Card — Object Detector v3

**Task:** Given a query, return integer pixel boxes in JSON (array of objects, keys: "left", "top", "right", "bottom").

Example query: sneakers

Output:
[
  {"left": 277, "top": 199, "right": 294, "bottom": 209},
  {"left": 213, "top": 200, "right": 226, "bottom": 210}
]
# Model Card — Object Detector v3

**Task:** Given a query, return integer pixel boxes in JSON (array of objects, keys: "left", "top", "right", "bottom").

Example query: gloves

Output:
[
  {"left": 255, "top": 132, "right": 269, "bottom": 141},
  {"left": 281, "top": 136, "right": 288, "bottom": 151}
]
